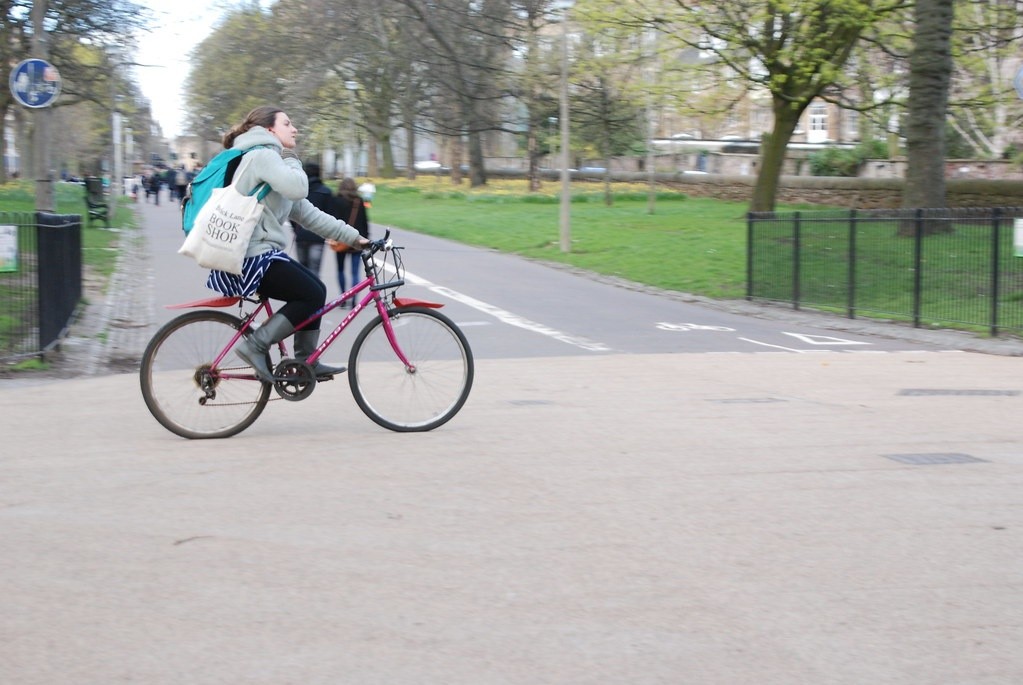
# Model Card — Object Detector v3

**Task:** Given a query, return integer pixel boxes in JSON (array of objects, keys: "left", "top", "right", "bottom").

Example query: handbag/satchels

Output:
[
  {"left": 328, "top": 238, "right": 351, "bottom": 251},
  {"left": 178, "top": 150, "right": 273, "bottom": 275}
]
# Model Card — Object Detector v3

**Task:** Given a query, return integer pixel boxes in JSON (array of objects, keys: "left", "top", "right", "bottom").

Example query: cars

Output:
[
  {"left": 721, "top": 132, "right": 744, "bottom": 140},
  {"left": 674, "top": 132, "right": 693, "bottom": 138}
]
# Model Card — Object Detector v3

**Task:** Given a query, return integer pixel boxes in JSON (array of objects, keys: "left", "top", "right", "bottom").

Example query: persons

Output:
[
  {"left": 137, "top": 153, "right": 201, "bottom": 204},
  {"left": 335, "top": 176, "right": 367, "bottom": 309},
  {"left": 205, "top": 106, "right": 372, "bottom": 385},
  {"left": 358, "top": 179, "right": 377, "bottom": 202},
  {"left": 294, "top": 162, "right": 334, "bottom": 273}
]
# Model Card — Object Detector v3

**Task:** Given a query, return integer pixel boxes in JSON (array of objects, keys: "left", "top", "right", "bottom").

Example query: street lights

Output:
[
  {"left": 112, "top": 96, "right": 121, "bottom": 196},
  {"left": 551, "top": 1, "right": 575, "bottom": 249},
  {"left": 346, "top": 79, "right": 358, "bottom": 184}
]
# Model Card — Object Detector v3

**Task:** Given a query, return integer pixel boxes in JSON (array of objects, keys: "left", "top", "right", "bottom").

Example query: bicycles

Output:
[{"left": 139, "top": 227, "right": 475, "bottom": 432}]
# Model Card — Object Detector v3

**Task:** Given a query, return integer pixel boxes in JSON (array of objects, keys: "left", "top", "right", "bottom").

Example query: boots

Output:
[
  {"left": 295, "top": 329, "right": 346, "bottom": 378},
  {"left": 234, "top": 313, "right": 294, "bottom": 384}
]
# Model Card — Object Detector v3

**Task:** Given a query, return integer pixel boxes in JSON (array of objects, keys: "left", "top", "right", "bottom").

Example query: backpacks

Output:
[{"left": 182, "top": 145, "right": 271, "bottom": 234}]
